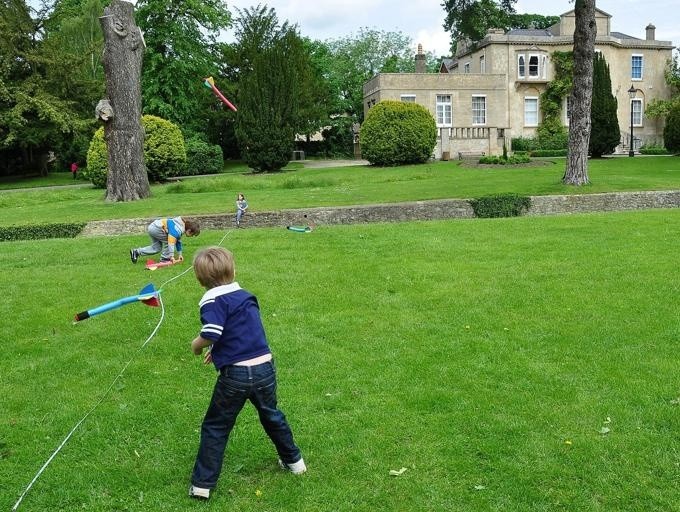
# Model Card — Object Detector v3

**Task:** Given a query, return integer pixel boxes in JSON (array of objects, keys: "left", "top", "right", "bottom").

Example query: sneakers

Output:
[
  {"left": 130, "top": 249, "right": 140, "bottom": 264},
  {"left": 188, "top": 484, "right": 210, "bottom": 500},
  {"left": 159, "top": 257, "right": 171, "bottom": 262},
  {"left": 277, "top": 457, "right": 307, "bottom": 475}
]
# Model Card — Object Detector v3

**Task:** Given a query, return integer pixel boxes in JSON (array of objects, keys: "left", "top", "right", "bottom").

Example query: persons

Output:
[
  {"left": 188, "top": 246, "right": 306, "bottom": 499},
  {"left": 130, "top": 216, "right": 200, "bottom": 264},
  {"left": 236, "top": 193, "right": 248, "bottom": 227},
  {"left": 70, "top": 162, "right": 78, "bottom": 180}
]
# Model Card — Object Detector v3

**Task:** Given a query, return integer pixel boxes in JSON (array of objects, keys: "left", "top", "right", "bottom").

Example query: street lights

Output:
[{"left": 627, "top": 83, "right": 637, "bottom": 157}]
